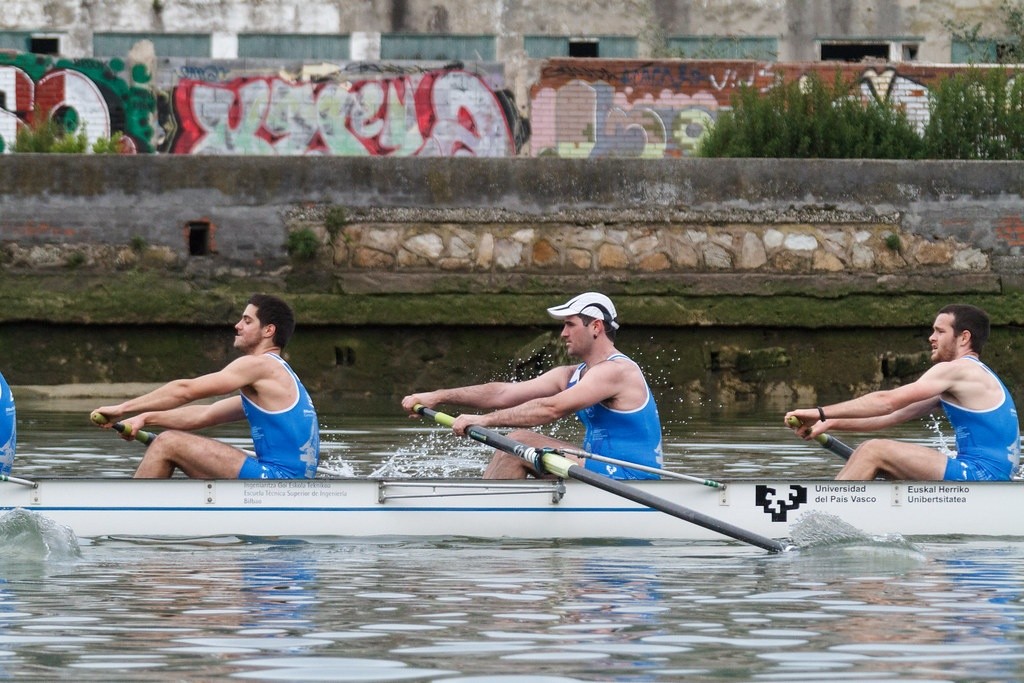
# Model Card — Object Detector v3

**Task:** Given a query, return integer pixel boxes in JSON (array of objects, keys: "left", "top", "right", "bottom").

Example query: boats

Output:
[{"left": 0, "top": 479, "right": 1024, "bottom": 543}]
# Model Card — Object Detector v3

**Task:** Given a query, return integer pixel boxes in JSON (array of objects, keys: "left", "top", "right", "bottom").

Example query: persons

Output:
[
  {"left": 784, "top": 304, "right": 1020, "bottom": 482},
  {"left": 0, "top": 373, "right": 17, "bottom": 476},
  {"left": 400, "top": 292, "right": 664, "bottom": 480},
  {"left": 89, "top": 293, "right": 319, "bottom": 480}
]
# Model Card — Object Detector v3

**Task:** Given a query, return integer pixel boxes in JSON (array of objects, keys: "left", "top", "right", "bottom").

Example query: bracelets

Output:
[{"left": 818, "top": 407, "right": 825, "bottom": 422}]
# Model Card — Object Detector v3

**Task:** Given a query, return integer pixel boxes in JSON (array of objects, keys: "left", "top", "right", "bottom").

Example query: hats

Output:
[{"left": 546, "top": 292, "right": 620, "bottom": 330}]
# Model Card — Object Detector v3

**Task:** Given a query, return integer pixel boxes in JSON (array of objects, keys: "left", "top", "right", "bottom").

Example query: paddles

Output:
[
  {"left": 787, "top": 415, "right": 888, "bottom": 479},
  {"left": 411, "top": 402, "right": 798, "bottom": 556},
  {"left": 94, "top": 412, "right": 158, "bottom": 447}
]
